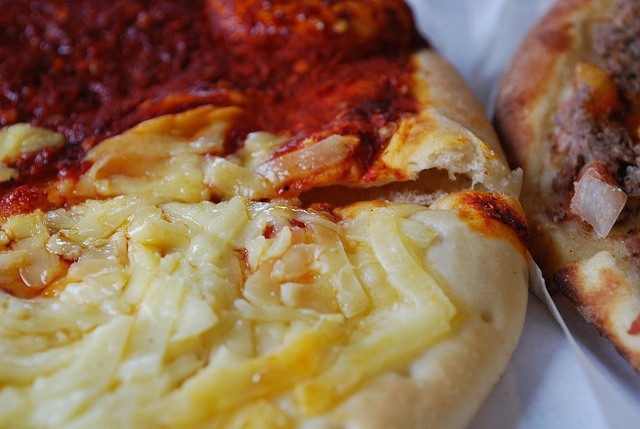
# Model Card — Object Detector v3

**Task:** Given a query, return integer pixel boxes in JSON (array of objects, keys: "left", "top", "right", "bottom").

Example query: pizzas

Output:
[
  {"left": 494, "top": 2, "right": 637, "bottom": 369},
  {"left": 0, "top": 1, "right": 531, "bottom": 428}
]
